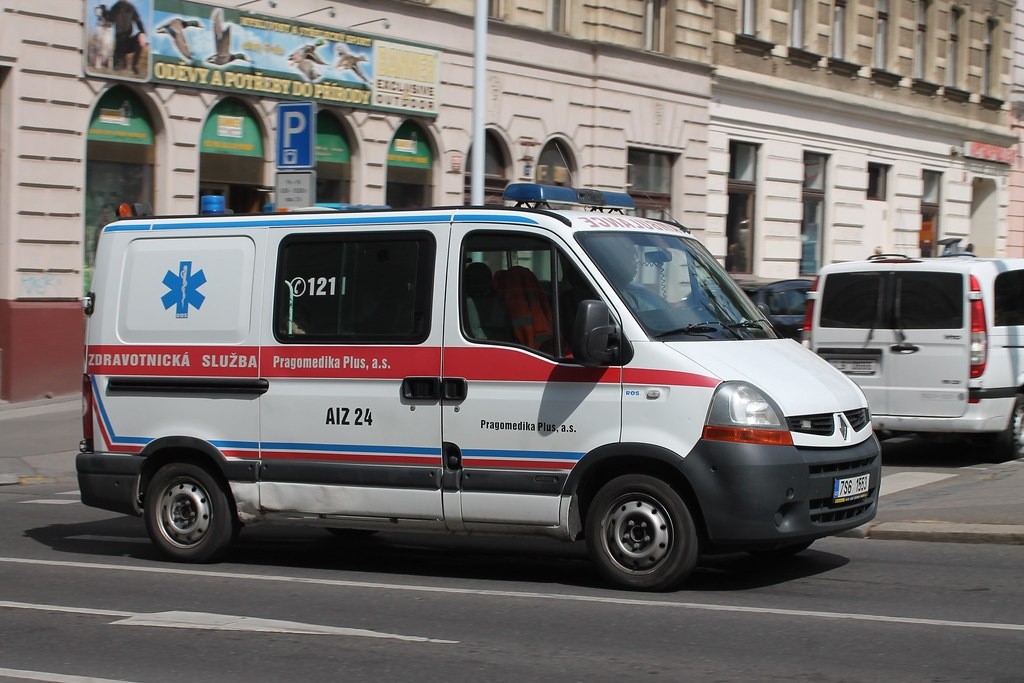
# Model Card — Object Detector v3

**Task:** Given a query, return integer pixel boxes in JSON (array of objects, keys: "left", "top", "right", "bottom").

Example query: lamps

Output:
[
  {"left": 292, "top": 6, "right": 335, "bottom": 18},
  {"left": 348, "top": 18, "right": 390, "bottom": 30},
  {"left": 234, "top": 0, "right": 277, "bottom": 9}
]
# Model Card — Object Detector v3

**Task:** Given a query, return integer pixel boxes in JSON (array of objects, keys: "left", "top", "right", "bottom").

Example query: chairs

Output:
[{"left": 465, "top": 263, "right": 571, "bottom": 359}]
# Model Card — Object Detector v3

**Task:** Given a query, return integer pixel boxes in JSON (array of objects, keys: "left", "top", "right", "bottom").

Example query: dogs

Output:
[{"left": 89, "top": 7, "right": 116, "bottom": 72}]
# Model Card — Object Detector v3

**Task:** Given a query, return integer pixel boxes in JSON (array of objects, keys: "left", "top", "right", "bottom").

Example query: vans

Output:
[{"left": 802, "top": 252, "right": 1024, "bottom": 461}]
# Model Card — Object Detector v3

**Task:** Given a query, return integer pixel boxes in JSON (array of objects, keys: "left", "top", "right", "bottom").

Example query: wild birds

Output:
[{"left": 155, "top": 6, "right": 372, "bottom": 86}]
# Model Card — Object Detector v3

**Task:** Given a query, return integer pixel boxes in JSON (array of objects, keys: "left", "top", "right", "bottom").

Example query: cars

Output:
[{"left": 731, "top": 273, "right": 817, "bottom": 343}]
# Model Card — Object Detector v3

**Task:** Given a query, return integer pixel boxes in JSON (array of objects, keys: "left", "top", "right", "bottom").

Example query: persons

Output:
[
  {"left": 100, "top": 0, "right": 145, "bottom": 74},
  {"left": 561, "top": 240, "right": 663, "bottom": 360},
  {"left": 93, "top": 204, "right": 111, "bottom": 260}
]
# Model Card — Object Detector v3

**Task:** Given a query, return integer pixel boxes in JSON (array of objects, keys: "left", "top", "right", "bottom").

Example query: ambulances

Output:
[{"left": 74, "top": 182, "right": 883, "bottom": 593}]
